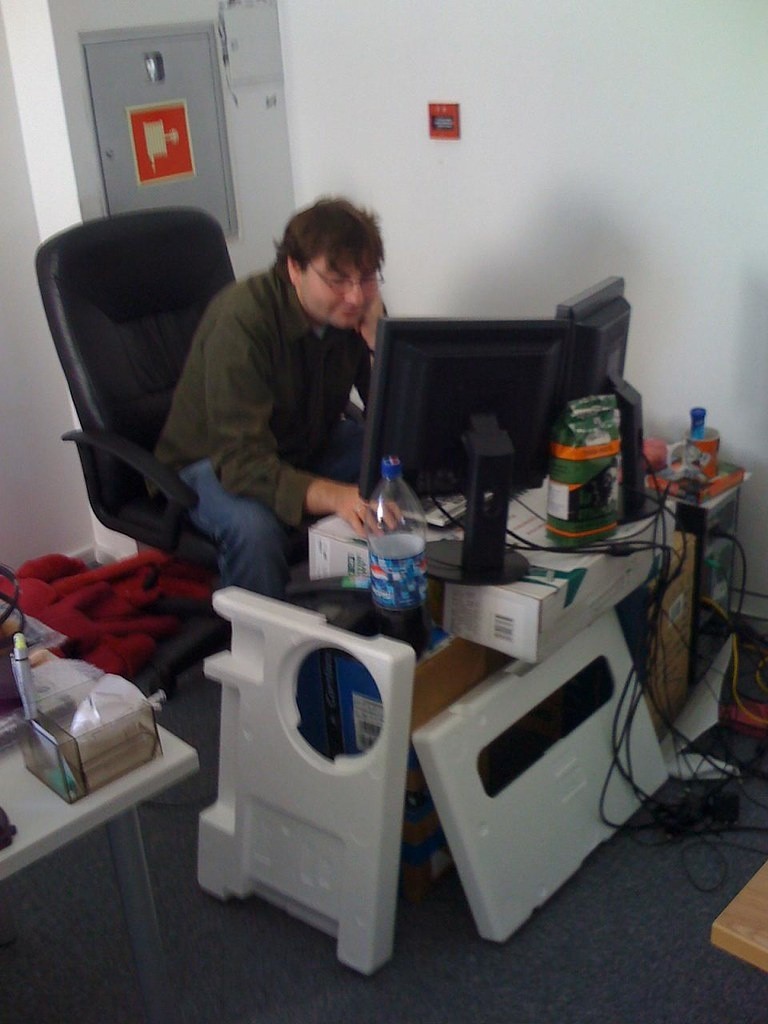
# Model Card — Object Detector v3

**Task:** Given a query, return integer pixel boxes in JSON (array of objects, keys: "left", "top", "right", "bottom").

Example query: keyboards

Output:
[{"left": 399, "top": 490, "right": 494, "bottom": 528}]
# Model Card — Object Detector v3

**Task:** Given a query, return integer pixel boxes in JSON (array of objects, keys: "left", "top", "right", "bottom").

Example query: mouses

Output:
[{"left": 378, "top": 515, "right": 411, "bottom": 534}]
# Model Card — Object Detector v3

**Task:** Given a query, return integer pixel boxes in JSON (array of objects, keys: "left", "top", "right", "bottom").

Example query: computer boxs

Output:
[{"left": 676, "top": 486, "right": 740, "bottom": 686}]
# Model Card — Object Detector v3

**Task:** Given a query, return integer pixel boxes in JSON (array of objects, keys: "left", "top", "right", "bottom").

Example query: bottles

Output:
[{"left": 367, "top": 456, "right": 429, "bottom": 662}]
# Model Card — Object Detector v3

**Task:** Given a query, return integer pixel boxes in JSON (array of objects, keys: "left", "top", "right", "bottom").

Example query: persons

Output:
[{"left": 146, "top": 197, "right": 403, "bottom": 600}]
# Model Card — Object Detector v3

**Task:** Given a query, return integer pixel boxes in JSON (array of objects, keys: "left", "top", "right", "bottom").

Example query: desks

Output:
[
  {"left": 304, "top": 465, "right": 746, "bottom": 671},
  {"left": 1, "top": 647, "right": 200, "bottom": 969}
]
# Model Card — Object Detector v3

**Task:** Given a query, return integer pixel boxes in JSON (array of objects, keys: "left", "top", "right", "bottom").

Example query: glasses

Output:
[{"left": 307, "top": 262, "right": 387, "bottom": 294}]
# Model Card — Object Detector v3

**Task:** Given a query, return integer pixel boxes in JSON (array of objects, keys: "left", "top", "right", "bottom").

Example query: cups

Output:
[{"left": 684, "top": 427, "right": 719, "bottom": 479}]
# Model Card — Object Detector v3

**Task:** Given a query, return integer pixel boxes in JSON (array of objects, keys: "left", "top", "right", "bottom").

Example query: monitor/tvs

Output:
[
  {"left": 558, "top": 275, "right": 667, "bottom": 524},
  {"left": 358, "top": 316, "right": 580, "bottom": 585}
]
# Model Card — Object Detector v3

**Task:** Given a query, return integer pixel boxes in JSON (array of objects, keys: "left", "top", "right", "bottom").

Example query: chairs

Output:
[{"left": 33, "top": 201, "right": 359, "bottom": 575}]
